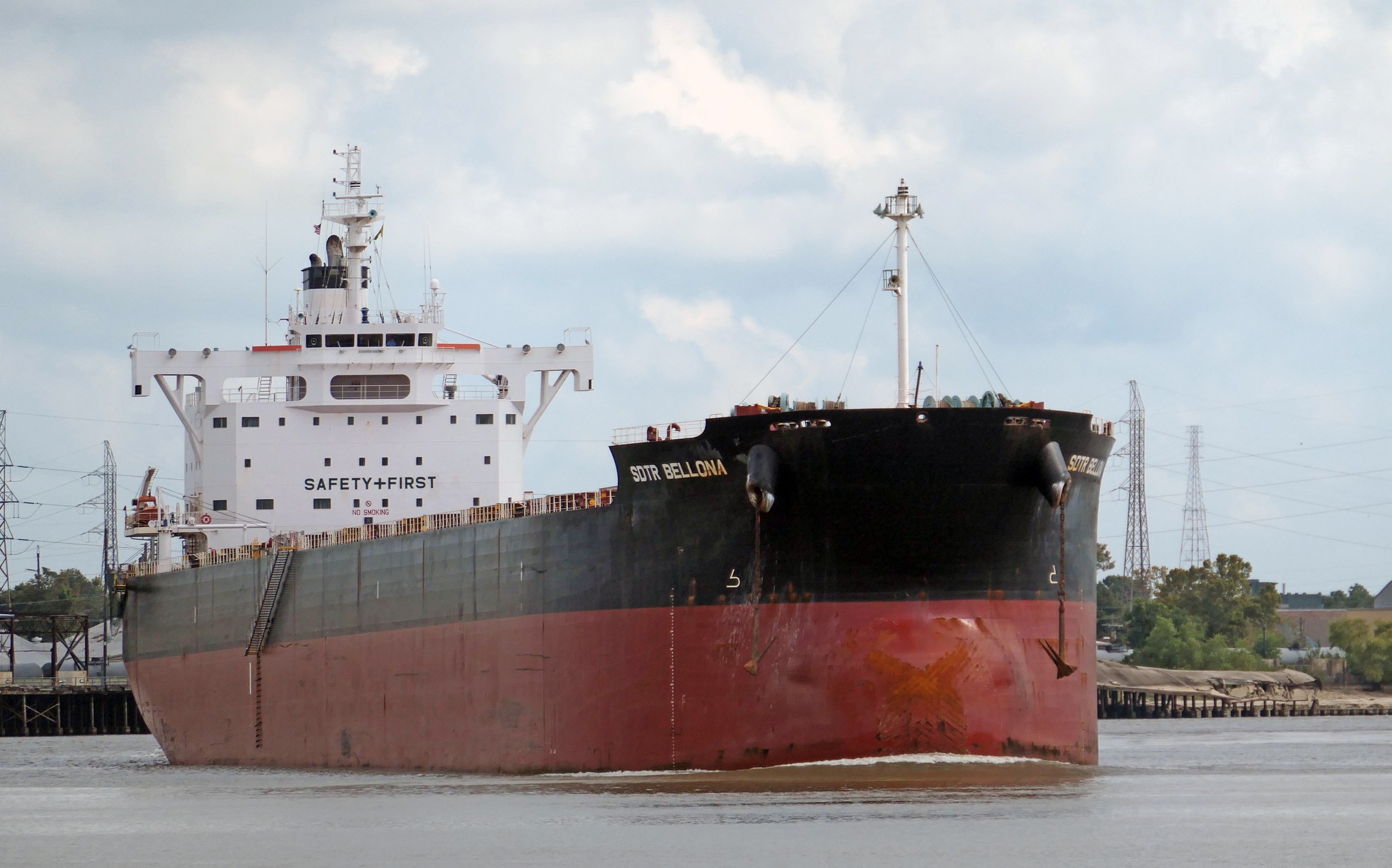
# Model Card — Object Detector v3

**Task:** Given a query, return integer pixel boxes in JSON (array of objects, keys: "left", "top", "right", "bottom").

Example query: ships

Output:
[{"left": 111, "top": 141, "right": 1118, "bottom": 773}]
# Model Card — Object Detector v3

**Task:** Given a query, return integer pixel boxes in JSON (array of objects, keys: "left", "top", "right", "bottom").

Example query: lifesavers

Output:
[{"left": 201, "top": 514, "right": 212, "bottom": 524}]
